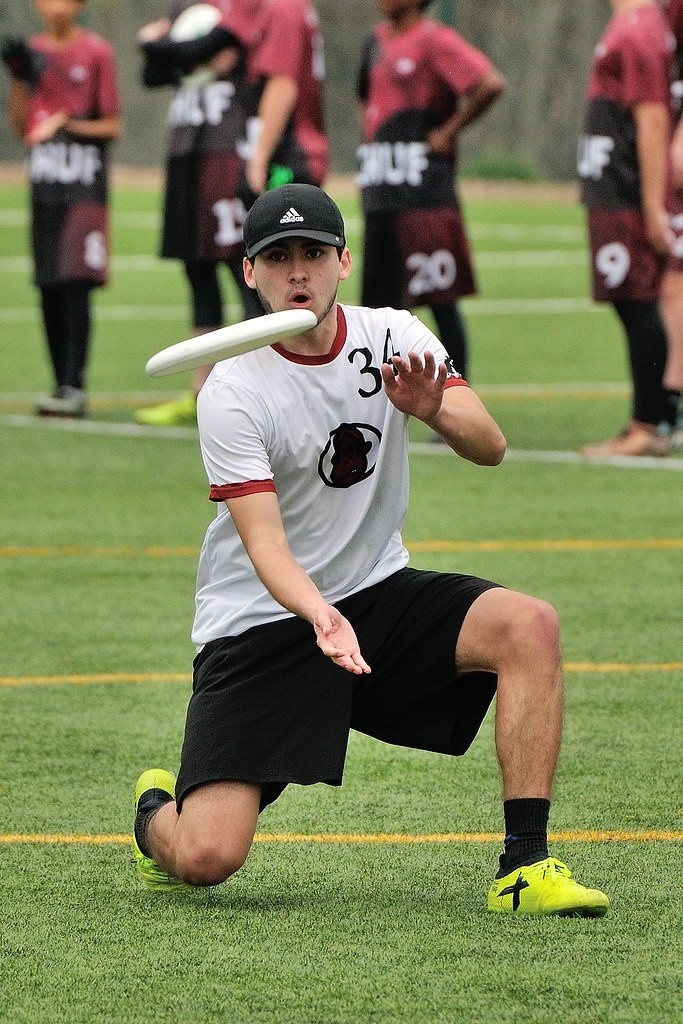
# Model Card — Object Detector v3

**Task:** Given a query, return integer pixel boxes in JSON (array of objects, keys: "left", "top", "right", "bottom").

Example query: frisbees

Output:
[{"left": 147, "top": 306, "right": 318, "bottom": 376}]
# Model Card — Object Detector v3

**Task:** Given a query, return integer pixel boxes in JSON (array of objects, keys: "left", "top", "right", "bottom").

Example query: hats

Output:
[{"left": 243, "top": 184, "right": 345, "bottom": 258}]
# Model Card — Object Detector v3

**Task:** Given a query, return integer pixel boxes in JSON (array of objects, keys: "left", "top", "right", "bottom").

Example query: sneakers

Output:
[
  {"left": 134, "top": 389, "right": 197, "bottom": 427},
  {"left": 488, "top": 854, "right": 609, "bottom": 918},
  {"left": 131, "top": 769, "right": 192, "bottom": 893}
]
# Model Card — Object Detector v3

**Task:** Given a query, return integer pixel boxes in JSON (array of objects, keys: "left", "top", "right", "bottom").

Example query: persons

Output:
[
  {"left": 576, "top": 1, "right": 683, "bottom": 454},
  {"left": 0, "top": 0, "right": 126, "bottom": 419},
  {"left": 354, "top": 1, "right": 509, "bottom": 444},
  {"left": 130, "top": 185, "right": 611, "bottom": 917},
  {"left": 133, "top": 1, "right": 326, "bottom": 426}
]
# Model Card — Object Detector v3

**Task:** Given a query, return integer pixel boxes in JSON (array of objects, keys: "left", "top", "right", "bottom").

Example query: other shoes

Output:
[
  {"left": 581, "top": 421, "right": 670, "bottom": 457},
  {"left": 38, "top": 386, "right": 87, "bottom": 415}
]
